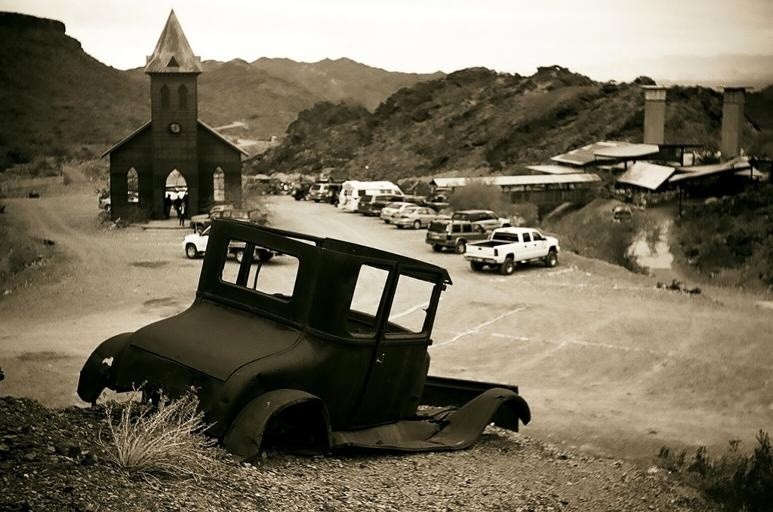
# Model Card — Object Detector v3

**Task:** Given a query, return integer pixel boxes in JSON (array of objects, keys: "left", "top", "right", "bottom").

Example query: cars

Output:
[
  {"left": 381, "top": 202, "right": 415, "bottom": 223},
  {"left": 611, "top": 203, "right": 633, "bottom": 226},
  {"left": 97, "top": 191, "right": 137, "bottom": 213},
  {"left": 391, "top": 207, "right": 450, "bottom": 229}
]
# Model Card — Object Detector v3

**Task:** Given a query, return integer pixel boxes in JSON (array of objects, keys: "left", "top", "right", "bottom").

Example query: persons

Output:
[{"left": 165, "top": 195, "right": 188, "bottom": 226}]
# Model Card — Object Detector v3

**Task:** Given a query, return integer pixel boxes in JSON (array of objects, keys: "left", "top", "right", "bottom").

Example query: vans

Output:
[{"left": 244, "top": 175, "right": 283, "bottom": 196}]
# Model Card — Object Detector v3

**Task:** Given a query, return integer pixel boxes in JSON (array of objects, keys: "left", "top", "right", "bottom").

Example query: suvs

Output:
[
  {"left": 451, "top": 209, "right": 510, "bottom": 231},
  {"left": 284, "top": 176, "right": 412, "bottom": 217},
  {"left": 423, "top": 219, "right": 490, "bottom": 254}
]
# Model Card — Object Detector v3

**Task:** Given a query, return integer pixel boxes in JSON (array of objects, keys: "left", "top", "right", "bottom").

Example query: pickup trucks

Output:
[
  {"left": 463, "top": 225, "right": 562, "bottom": 274},
  {"left": 177, "top": 217, "right": 284, "bottom": 264}
]
